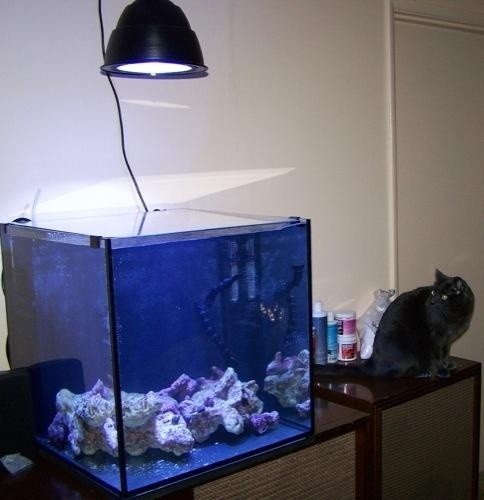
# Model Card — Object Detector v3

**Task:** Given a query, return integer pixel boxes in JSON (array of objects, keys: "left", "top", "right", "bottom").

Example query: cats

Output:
[{"left": 312, "top": 268, "right": 475, "bottom": 381}]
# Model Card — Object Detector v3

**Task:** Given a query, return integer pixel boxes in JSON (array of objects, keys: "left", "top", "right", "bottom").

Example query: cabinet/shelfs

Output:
[
  {"left": 312, "top": 345, "right": 481, "bottom": 499},
  {"left": 172, "top": 396, "right": 370, "bottom": 499}
]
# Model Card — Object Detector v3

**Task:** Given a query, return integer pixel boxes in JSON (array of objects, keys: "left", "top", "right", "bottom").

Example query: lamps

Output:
[{"left": 95, "top": 0, "right": 212, "bottom": 81}]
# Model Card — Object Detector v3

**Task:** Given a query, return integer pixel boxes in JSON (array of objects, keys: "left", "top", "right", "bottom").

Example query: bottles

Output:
[
  {"left": 314, "top": 378, "right": 329, "bottom": 422},
  {"left": 311, "top": 300, "right": 327, "bottom": 368},
  {"left": 328, "top": 382, "right": 338, "bottom": 415},
  {"left": 325, "top": 311, "right": 338, "bottom": 365}
]
